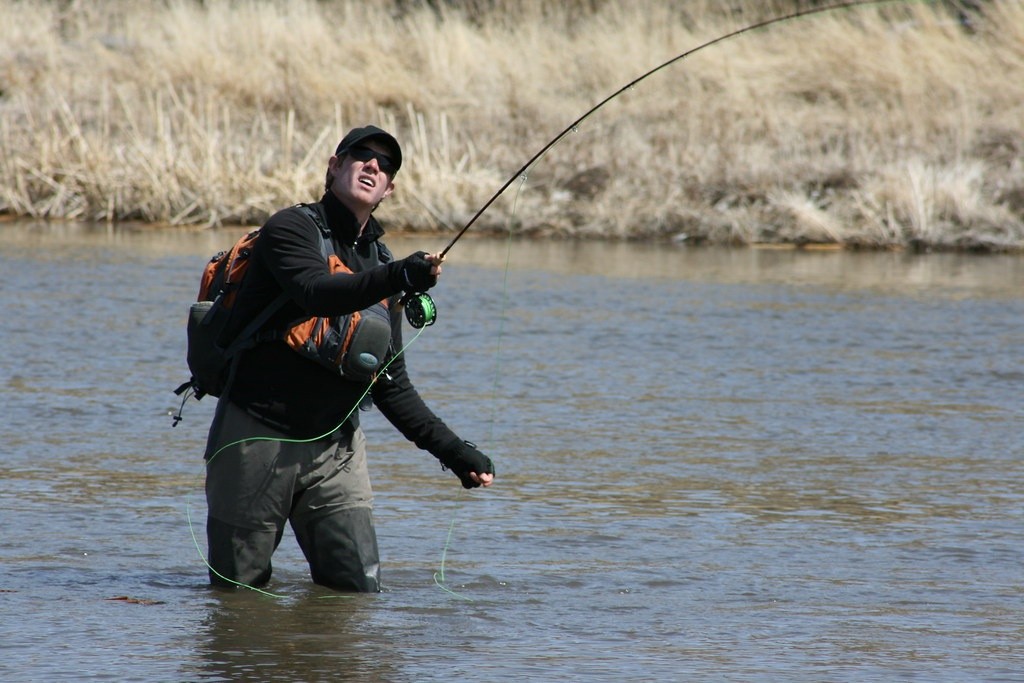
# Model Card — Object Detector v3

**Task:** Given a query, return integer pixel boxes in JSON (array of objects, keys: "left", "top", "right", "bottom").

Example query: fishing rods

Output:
[{"left": 391, "top": 0, "right": 884, "bottom": 332}]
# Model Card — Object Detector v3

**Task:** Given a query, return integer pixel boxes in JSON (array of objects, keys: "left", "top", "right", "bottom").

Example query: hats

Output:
[{"left": 336, "top": 125, "right": 401, "bottom": 174}]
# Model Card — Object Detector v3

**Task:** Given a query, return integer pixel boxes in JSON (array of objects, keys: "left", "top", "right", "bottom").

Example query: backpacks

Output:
[{"left": 174, "top": 203, "right": 391, "bottom": 401}]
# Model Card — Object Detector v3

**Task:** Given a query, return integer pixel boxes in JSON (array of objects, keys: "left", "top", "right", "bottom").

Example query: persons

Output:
[{"left": 202, "top": 125, "right": 496, "bottom": 592}]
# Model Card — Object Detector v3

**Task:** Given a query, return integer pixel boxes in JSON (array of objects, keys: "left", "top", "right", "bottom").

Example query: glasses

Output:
[{"left": 337, "top": 145, "right": 397, "bottom": 182}]
